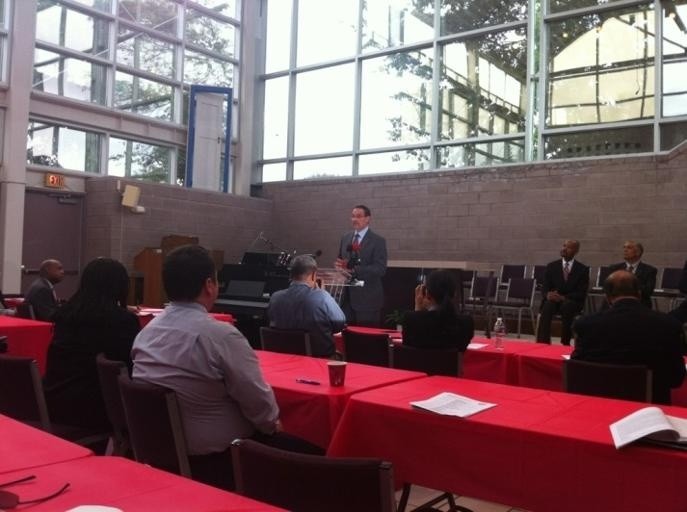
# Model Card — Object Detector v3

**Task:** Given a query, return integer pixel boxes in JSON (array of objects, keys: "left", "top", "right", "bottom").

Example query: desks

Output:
[
  {"left": 2, "top": 298, "right": 25, "bottom": 309},
  {"left": 350, "top": 376, "right": 687, "bottom": 512},
  {"left": 316, "top": 270, "right": 351, "bottom": 310},
  {"left": 0, "top": 314, "right": 54, "bottom": 376},
  {"left": 209, "top": 298, "right": 270, "bottom": 350},
  {"left": 251, "top": 349, "right": 427, "bottom": 451},
  {"left": 136, "top": 306, "right": 234, "bottom": 330},
  {"left": 463, "top": 336, "right": 687, "bottom": 405},
  {"left": 333, "top": 326, "right": 403, "bottom": 354},
  {"left": 386, "top": 259, "right": 502, "bottom": 312}
]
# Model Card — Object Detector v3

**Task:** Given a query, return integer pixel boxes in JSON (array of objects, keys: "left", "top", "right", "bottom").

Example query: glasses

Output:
[
  {"left": 351, "top": 214, "right": 366, "bottom": 219},
  {"left": 0, "top": 476, "right": 69, "bottom": 508}
]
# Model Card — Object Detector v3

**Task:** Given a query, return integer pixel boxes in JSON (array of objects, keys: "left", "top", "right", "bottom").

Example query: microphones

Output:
[{"left": 345, "top": 243, "right": 352, "bottom": 262}]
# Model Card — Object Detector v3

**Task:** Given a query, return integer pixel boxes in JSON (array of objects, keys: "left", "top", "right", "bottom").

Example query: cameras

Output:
[
  {"left": 422, "top": 285, "right": 427, "bottom": 297},
  {"left": 316, "top": 279, "right": 322, "bottom": 288}
]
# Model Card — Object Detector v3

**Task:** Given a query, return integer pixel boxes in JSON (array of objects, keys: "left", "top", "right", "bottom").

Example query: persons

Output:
[
  {"left": 397, "top": 269, "right": 475, "bottom": 378},
  {"left": 132, "top": 245, "right": 327, "bottom": 483},
  {"left": 334, "top": 205, "right": 387, "bottom": 362},
  {"left": 600, "top": 240, "right": 658, "bottom": 312},
  {"left": 536, "top": 240, "right": 590, "bottom": 345},
  {"left": 570, "top": 269, "right": 686, "bottom": 405},
  {"left": 23, "top": 260, "right": 67, "bottom": 322},
  {"left": 668, "top": 260, "right": 687, "bottom": 325},
  {"left": 41, "top": 256, "right": 142, "bottom": 432},
  {"left": 265, "top": 254, "right": 346, "bottom": 360}
]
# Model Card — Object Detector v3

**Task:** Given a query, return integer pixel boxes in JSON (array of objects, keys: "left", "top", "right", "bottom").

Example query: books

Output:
[
  {"left": 608, "top": 407, "right": 687, "bottom": 453},
  {"left": 409, "top": 392, "right": 495, "bottom": 419}
]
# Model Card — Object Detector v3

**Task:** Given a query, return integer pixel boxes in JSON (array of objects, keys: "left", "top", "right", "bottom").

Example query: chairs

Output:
[
  {"left": 493, "top": 278, "right": 536, "bottom": 339},
  {"left": 497, "top": 264, "right": 527, "bottom": 317},
  {"left": 0, "top": 354, "right": 52, "bottom": 434},
  {"left": 95, "top": 351, "right": 133, "bottom": 460},
  {"left": 532, "top": 265, "right": 547, "bottom": 291},
  {"left": 260, "top": 326, "right": 312, "bottom": 356},
  {"left": 388, "top": 344, "right": 463, "bottom": 377},
  {"left": 652, "top": 267, "right": 684, "bottom": 311},
  {"left": 118, "top": 368, "right": 191, "bottom": 478},
  {"left": 592, "top": 266, "right": 611, "bottom": 309},
  {"left": 564, "top": 358, "right": 652, "bottom": 403},
  {"left": 466, "top": 277, "right": 499, "bottom": 339},
  {"left": 15, "top": 304, "right": 35, "bottom": 319},
  {"left": 536, "top": 311, "right": 583, "bottom": 342},
  {"left": 422, "top": 267, "right": 433, "bottom": 286},
  {"left": 461, "top": 269, "right": 477, "bottom": 301},
  {"left": 341, "top": 323, "right": 390, "bottom": 367},
  {"left": 231, "top": 439, "right": 441, "bottom": 512}
]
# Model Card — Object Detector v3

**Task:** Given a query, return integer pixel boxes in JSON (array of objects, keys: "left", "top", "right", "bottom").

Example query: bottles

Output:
[{"left": 494, "top": 317, "right": 506, "bottom": 351}]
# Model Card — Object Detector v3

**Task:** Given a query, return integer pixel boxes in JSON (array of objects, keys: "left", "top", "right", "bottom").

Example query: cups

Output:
[{"left": 328, "top": 361, "right": 346, "bottom": 387}]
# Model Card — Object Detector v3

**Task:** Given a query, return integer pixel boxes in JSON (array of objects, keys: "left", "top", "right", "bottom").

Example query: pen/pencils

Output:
[{"left": 297, "top": 379, "right": 321, "bottom": 385}]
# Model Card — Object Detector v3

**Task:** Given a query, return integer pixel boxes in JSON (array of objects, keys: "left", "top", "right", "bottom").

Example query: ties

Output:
[
  {"left": 352, "top": 234, "right": 359, "bottom": 253},
  {"left": 563, "top": 262, "right": 569, "bottom": 282},
  {"left": 628, "top": 265, "right": 634, "bottom": 273}
]
